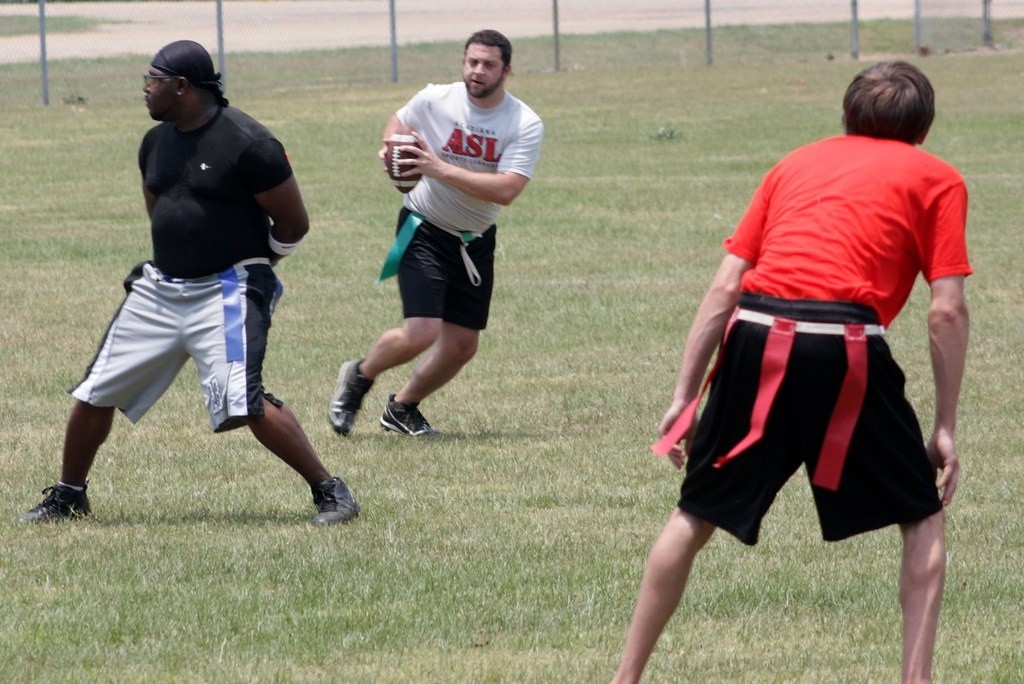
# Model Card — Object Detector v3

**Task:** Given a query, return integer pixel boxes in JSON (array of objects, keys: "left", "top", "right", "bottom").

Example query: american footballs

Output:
[{"left": 385, "top": 125, "right": 423, "bottom": 195}]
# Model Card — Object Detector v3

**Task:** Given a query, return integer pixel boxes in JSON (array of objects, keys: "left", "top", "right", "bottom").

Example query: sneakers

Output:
[
  {"left": 328, "top": 357, "right": 374, "bottom": 435},
  {"left": 311, "top": 477, "right": 360, "bottom": 527},
  {"left": 15, "top": 478, "right": 93, "bottom": 523},
  {"left": 379, "top": 395, "right": 436, "bottom": 435}
]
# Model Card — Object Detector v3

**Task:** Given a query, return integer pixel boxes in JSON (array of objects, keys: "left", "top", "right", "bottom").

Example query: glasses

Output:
[{"left": 142, "top": 74, "right": 193, "bottom": 90}]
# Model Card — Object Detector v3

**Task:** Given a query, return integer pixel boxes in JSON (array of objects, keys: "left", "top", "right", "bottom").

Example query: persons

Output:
[
  {"left": 610, "top": 63, "right": 973, "bottom": 683},
  {"left": 331, "top": 30, "right": 543, "bottom": 437},
  {"left": 17, "top": 40, "right": 359, "bottom": 525}
]
